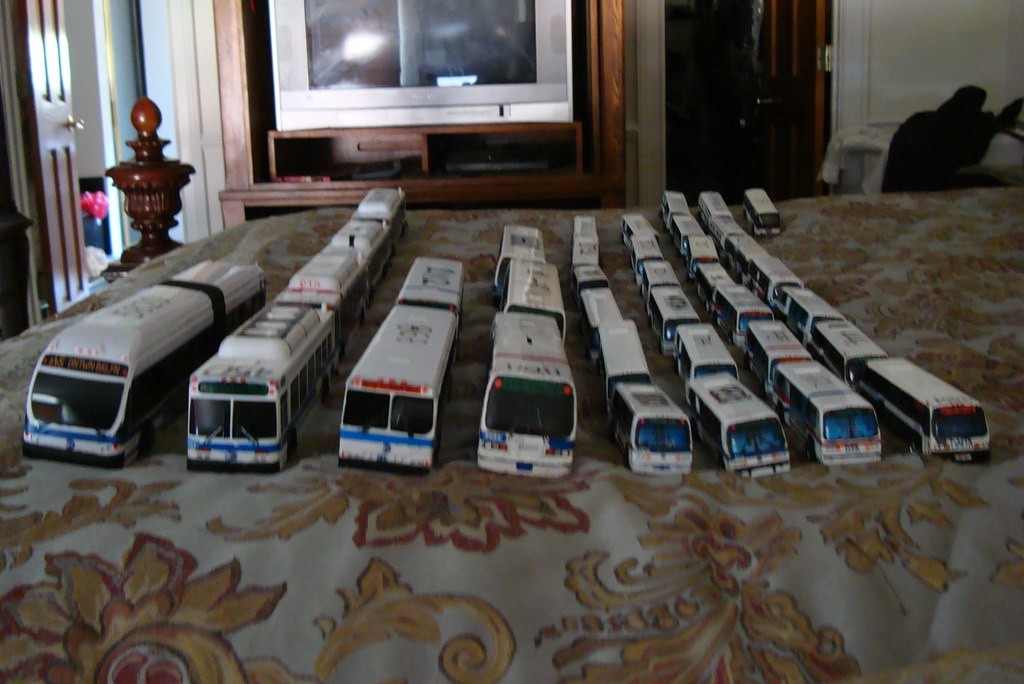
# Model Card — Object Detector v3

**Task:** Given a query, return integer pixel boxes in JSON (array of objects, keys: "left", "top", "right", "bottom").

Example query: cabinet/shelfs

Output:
[{"left": 212, "top": 0, "right": 626, "bottom": 230}]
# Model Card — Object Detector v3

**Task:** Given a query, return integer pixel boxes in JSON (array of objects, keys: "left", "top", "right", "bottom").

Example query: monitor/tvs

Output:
[{"left": 269, "top": 0, "right": 575, "bottom": 132}]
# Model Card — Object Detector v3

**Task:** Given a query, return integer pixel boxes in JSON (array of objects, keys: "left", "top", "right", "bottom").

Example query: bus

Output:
[
  {"left": 658, "top": 190, "right": 812, "bottom": 383},
  {"left": 743, "top": 189, "right": 782, "bottom": 236},
  {"left": 769, "top": 361, "right": 883, "bottom": 467},
  {"left": 609, "top": 383, "right": 695, "bottom": 475},
  {"left": 858, "top": 356, "right": 991, "bottom": 465},
  {"left": 572, "top": 214, "right": 651, "bottom": 399},
  {"left": 477, "top": 225, "right": 578, "bottom": 480},
  {"left": 687, "top": 373, "right": 792, "bottom": 481},
  {"left": 620, "top": 216, "right": 740, "bottom": 381},
  {"left": 698, "top": 190, "right": 887, "bottom": 387}
]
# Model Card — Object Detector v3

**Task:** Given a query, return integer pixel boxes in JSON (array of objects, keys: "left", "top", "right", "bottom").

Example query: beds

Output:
[{"left": 0, "top": 185, "right": 1024, "bottom": 684}]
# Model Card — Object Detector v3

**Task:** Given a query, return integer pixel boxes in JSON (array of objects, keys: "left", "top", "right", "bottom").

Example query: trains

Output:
[
  {"left": 188, "top": 189, "right": 406, "bottom": 472},
  {"left": 338, "top": 256, "right": 467, "bottom": 471},
  {"left": 22, "top": 259, "right": 266, "bottom": 469}
]
